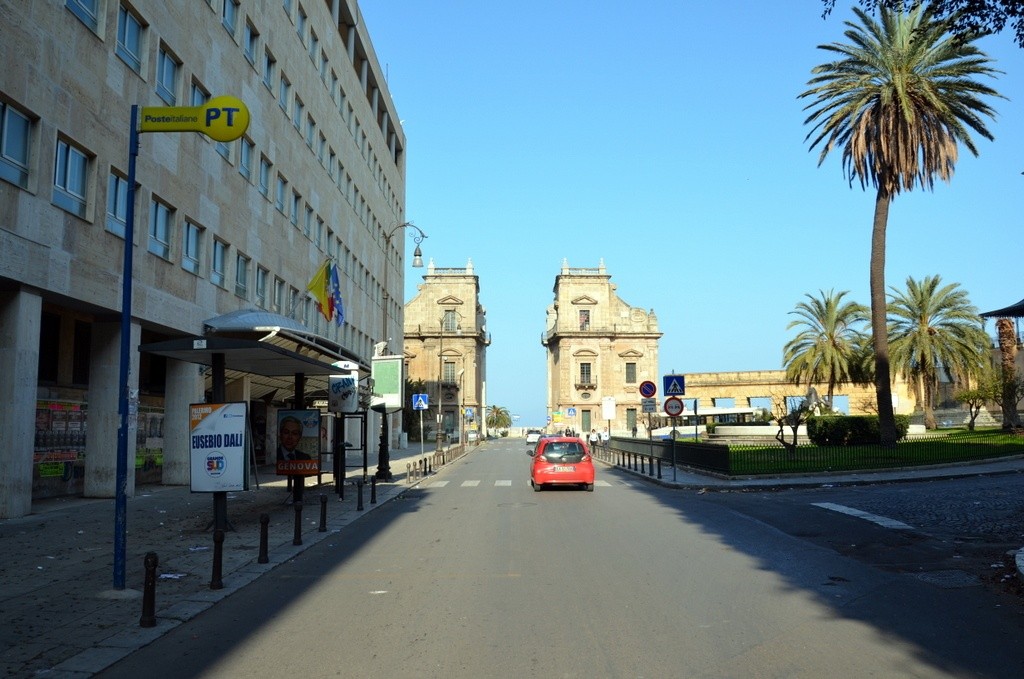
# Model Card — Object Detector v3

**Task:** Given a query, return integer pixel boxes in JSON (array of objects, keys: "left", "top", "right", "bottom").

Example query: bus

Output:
[{"left": 643, "top": 407, "right": 764, "bottom": 439}]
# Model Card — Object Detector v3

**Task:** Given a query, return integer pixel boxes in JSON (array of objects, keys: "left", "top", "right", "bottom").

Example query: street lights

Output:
[
  {"left": 376, "top": 223, "right": 428, "bottom": 479},
  {"left": 463, "top": 349, "right": 480, "bottom": 441},
  {"left": 435, "top": 307, "right": 465, "bottom": 456}
]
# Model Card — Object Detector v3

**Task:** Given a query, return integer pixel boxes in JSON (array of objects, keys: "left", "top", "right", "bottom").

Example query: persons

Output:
[
  {"left": 566, "top": 426, "right": 610, "bottom": 454},
  {"left": 632, "top": 424, "right": 637, "bottom": 437},
  {"left": 277, "top": 416, "right": 311, "bottom": 462}
]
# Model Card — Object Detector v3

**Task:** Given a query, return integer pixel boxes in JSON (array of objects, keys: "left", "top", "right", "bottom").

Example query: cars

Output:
[
  {"left": 525, "top": 430, "right": 542, "bottom": 445},
  {"left": 526, "top": 435, "right": 595, "bottom": 491},
  {"left": 466, "top": 429, "right": 480, "bottom": 441}
]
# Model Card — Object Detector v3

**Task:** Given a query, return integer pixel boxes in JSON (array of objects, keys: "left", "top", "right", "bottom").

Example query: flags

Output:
[
  {"left": 331, "top": 264, "right": 344, "bottom": 327},
  {"left": 307, "top": 258, "right": 334, "bottom": 322}
]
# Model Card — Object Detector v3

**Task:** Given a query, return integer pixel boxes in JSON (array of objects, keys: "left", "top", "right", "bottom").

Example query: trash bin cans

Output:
[{"left": 399, "top": 433, "right": 408, "bottom": 449}]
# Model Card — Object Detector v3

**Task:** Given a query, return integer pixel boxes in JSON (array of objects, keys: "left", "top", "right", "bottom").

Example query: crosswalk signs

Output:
[
  {"left": 412, "top": 393, "right": 428, "bottom": 410},
  {"left": 567, "top": 408, "right": 576, "bottom": 416},
  {"left": 663, "top": 376, "right": 685, "bottom": 395},
  {"left": 466, "top": 409, "right": 472, "bottom": 417}
]
